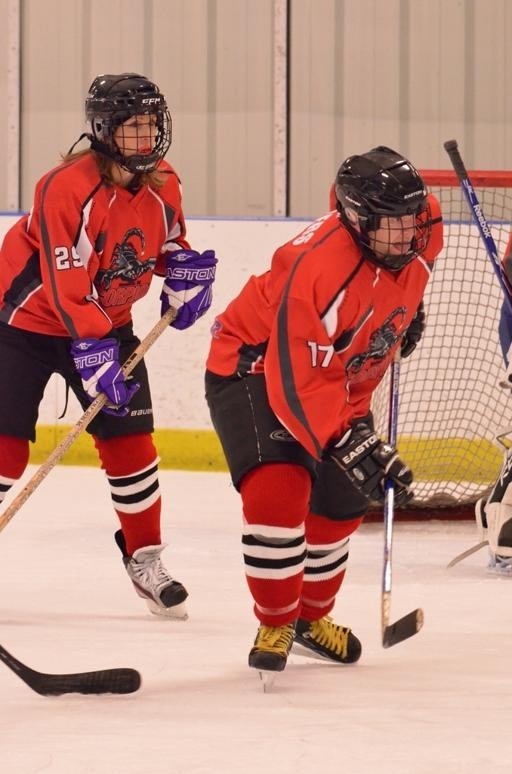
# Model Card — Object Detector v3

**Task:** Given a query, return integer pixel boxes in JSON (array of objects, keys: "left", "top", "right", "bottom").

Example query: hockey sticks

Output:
[
  {"left": 382, "top": 346, "right": 424, "bottom": 650},
  {"left": 0, "top": 644, "right": 141, "bottom": 696}
]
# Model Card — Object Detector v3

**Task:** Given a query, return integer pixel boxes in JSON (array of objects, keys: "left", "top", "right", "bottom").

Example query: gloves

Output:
[
  {"left": 329, "top": 423, "right": 413, "bottom": 510},
  {"left": 71, "top": 338, "right": 140, "bottom": 417},
  {"left": 159, "top": 250, "right": 219, "bottom": 330},
  {"left": 401, "top": 303, "right": 425, "bottom": 357}
]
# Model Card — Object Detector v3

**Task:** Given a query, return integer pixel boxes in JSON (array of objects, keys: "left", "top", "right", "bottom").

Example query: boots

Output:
[
  {"left": 112, "top": 528, "right": 189, "bottom": 608},
  {"left": 247, "top": 619, "right": 296, "bottom": 672},
  {"left": 294, "top": 616, "right": 362, "bottom": 663}
]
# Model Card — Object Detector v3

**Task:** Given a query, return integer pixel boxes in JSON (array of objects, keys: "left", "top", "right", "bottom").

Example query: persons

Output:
[
  {"left": 0, "top": 74, "right": 218, "bottom": 609},
  {"left": 474, "top": 218, "right": 512, "bottom": 557},
  {"left": 205, "top": 146, "right": 443, "bottom": 672}
]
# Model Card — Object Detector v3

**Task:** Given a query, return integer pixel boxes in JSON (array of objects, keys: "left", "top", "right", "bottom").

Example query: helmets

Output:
[
  {"left": 336, "top": 147, "right": 429, "bottom": 242},
  {"left": 84, "top": 73, "right": 166, "bottom": 143}
]
